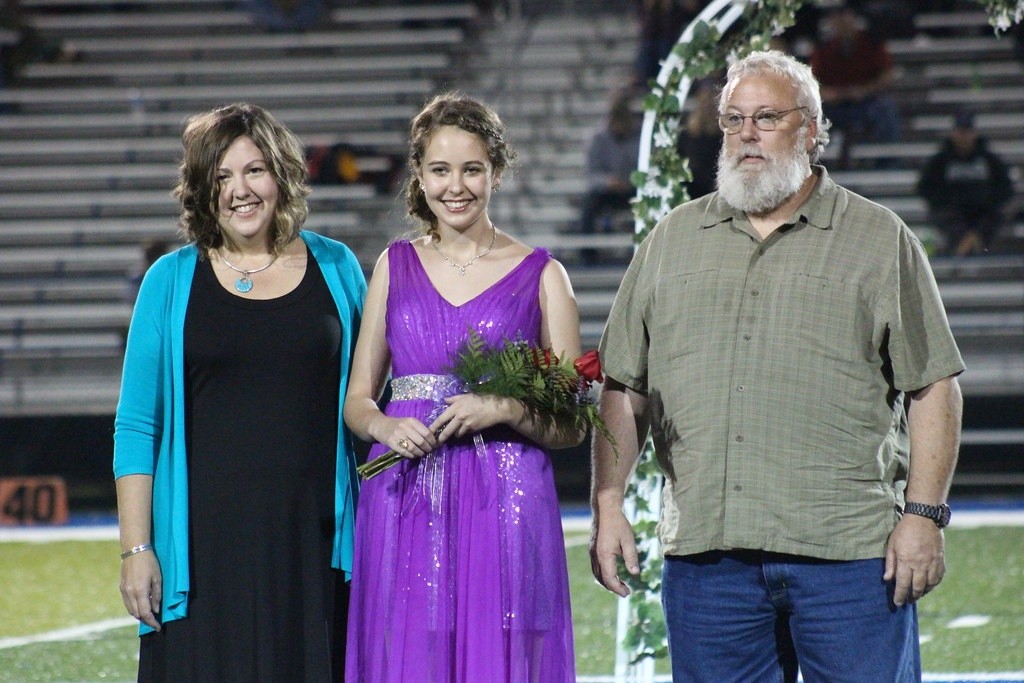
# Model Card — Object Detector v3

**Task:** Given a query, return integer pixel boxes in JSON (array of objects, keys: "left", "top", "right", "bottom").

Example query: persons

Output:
[
  {"left": 577, "top": 98, "right": 642, "bottom": 233},
  {"left": 342, "top": 93, "right": 585, "bottom": 683},
  {"left": 915, "top": 104, "right": 1015, "bottom": 257},
  {"left": 112, "top": 99, "right": 368, "bottom": 683},
  {"left": 674, "top": 80, "right": 726, "bottom": 197},
  {"left": 810, "top": 9, "right": 902, "bottom": 141},
  {"left": 585, "top": 46, "right": 966, "bottom": 683}
]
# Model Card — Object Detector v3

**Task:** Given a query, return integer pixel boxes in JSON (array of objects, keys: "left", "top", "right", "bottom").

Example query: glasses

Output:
[{"left": 716, "top": 106, "right": 809, "bottom": 131}]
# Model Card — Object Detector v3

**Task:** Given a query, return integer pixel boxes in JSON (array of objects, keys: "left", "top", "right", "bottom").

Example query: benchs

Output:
[{"left": 0, "top": 0, "right": 1024, "bottom": 489}]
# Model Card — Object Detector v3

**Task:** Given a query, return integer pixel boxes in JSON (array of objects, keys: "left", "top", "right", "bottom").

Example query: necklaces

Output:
[
  {"left": 215, "top": 246, "right": 279, "bottom": 294},
  {"left": 430, "top": 223, "right": 496, "bottom": 276}
]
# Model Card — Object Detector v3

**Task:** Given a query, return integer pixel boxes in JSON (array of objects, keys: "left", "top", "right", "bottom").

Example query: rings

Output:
[{"left": 398, "top": 437, "right": 408, "bottom": 449}]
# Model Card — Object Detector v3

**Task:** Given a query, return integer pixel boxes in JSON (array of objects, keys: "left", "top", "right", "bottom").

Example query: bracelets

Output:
[{"left": 120, "top": 542, "right": 153, "bottom": 558}]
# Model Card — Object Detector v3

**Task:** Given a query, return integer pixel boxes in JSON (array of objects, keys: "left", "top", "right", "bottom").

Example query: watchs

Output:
[{"left": 903, "top": 500, "right": 952, "bottom": 529}]
